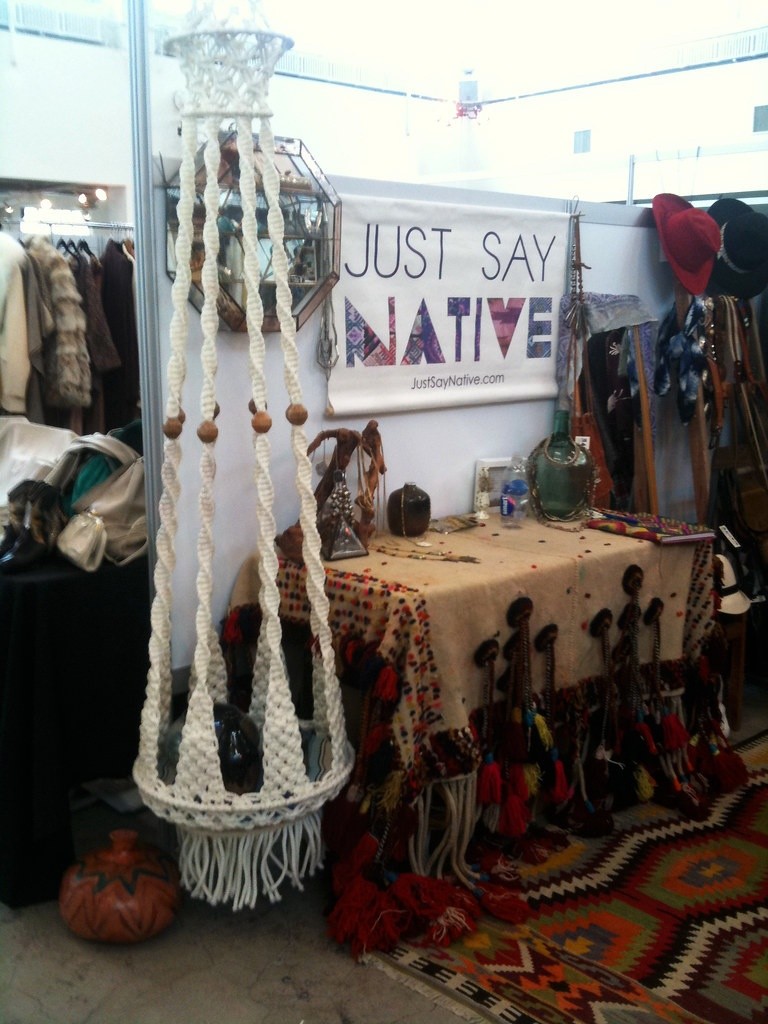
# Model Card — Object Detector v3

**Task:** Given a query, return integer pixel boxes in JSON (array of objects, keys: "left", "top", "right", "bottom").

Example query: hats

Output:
[
  {"left": 652, "top": 192, "right": 722, "bottom": 295},
  {"left": 707, "top": 198, "right": 768, "bottom": 299}
]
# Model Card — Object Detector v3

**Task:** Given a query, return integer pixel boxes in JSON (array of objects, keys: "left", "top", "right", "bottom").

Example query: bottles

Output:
[
  {"left": 527, "top": 409, "right": 594, "bottom": 526},
  {"left": 500, "top": 451, "right": 531, "bottom": 530},
  {"left": 387, "top": 481, "right": 430, "bottom": 537}
]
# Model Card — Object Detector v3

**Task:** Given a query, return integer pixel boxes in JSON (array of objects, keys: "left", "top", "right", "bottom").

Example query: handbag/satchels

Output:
[{"left": 56, "top": 509, "right": 107, "bottom": 571}]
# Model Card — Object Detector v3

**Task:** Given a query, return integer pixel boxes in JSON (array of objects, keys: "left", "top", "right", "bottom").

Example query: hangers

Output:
[{"left": 41, "top": 217, "right": 135, "bottom": 272}]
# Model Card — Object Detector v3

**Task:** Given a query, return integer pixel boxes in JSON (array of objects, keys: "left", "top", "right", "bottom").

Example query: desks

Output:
[{"left": 224, "top": 502, "right": 745, "bottom": 958}]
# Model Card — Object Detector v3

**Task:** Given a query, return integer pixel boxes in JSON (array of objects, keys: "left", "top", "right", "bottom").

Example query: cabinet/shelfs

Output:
[{"left": 160, "top": 133, "right": 340, "bottom": 335}]
